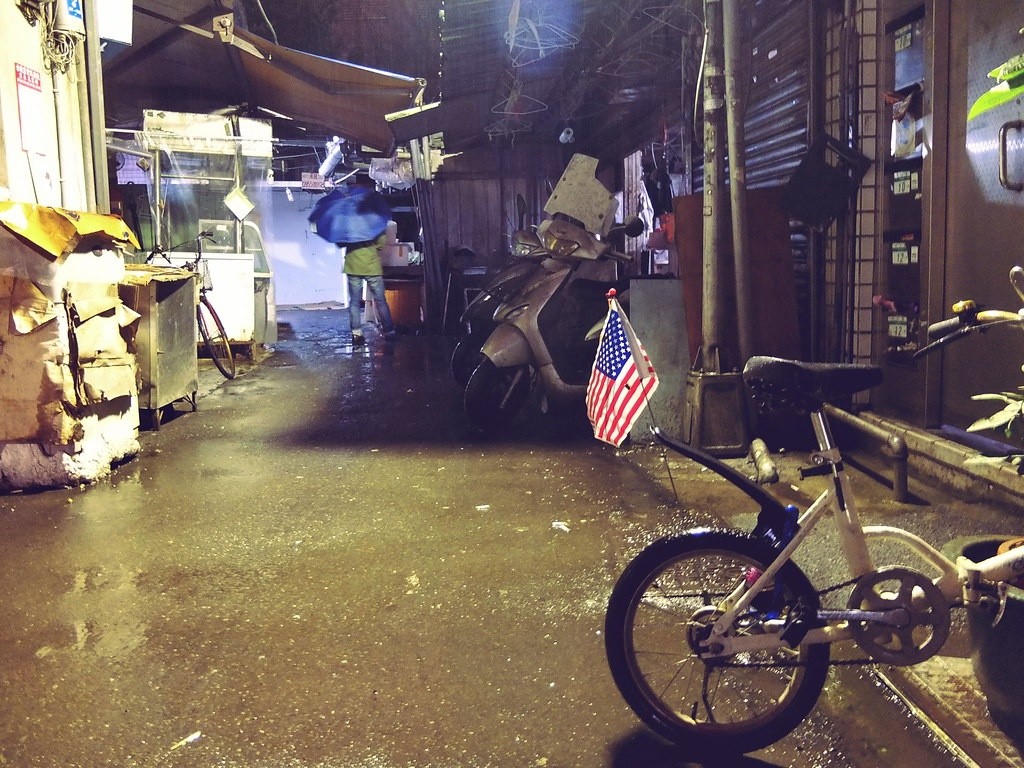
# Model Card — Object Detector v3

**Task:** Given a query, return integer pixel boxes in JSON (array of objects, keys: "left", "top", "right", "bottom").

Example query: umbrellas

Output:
[{"left": 307, "top": 184, "right": 391, "bottom": 243}]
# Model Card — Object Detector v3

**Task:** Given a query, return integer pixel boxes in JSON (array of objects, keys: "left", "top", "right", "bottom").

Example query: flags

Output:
[{"left": 585, "top": 296, "right": 659, "bottom": 448}]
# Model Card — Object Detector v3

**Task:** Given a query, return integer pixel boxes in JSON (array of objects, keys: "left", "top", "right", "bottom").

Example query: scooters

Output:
[
  {"left": 460, "top": 212, "right": 632, "bottom": 433},
  {"left": 448, "top": 195, "right": 676, "bottom": 389}
]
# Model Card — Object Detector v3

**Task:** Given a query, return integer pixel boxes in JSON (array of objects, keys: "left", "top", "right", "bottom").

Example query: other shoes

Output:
[
  {"left": 385, "top": 333, "right": 401, "bottom": 340},
  {"left": 352, "top": 333, "right": 366, "bottom": 345}
]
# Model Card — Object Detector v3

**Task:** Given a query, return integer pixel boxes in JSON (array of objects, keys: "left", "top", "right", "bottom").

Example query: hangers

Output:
[{"left": 484, "top": 5, "right": 704, "bottom": 137}]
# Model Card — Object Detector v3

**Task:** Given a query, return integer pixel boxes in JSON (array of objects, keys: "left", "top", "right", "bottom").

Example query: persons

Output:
[{"left": 337, "top": 233, "right": 399, "bottom": 345}]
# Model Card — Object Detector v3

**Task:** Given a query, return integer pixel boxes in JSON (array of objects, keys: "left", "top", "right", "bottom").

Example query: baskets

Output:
[{"left": 181, "top": 258, "right": 213, "bottom": 292}]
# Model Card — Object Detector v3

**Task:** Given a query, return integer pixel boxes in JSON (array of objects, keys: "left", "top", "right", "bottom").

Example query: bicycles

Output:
[
  {"left": 604, "top": 264, "right": 1024, "bottom": 755},
  {"left": 142, "top": 229, "right": 236, "bottom": 380}
]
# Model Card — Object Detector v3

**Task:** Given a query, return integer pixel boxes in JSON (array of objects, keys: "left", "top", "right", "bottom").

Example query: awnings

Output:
[{"left": 230, "top": 26, "right": 426, "bottom": 155}]
